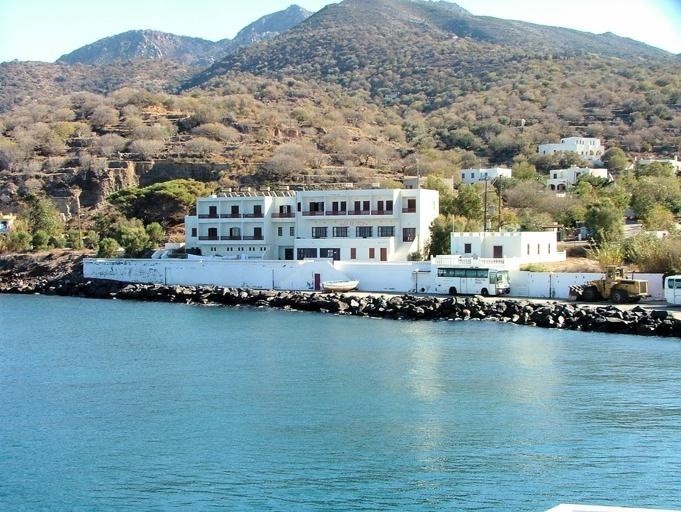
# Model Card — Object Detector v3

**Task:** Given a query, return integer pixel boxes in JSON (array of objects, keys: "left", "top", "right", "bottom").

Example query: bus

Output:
[
  {"left": 664, "top": 275, "right": 681, "bottom": 306},
  {"left": 435, "top": 265, "right": 512, "bottom": 297}
]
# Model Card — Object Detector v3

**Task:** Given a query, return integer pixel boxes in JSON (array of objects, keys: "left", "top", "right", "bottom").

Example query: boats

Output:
[{"left": 322, "top": 279, "right": 362, "bottom": 293}]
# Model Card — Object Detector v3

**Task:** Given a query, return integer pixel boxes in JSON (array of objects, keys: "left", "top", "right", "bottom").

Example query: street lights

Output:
[{"left": 68, "top": 183, "right": 84, "bottom": 251}]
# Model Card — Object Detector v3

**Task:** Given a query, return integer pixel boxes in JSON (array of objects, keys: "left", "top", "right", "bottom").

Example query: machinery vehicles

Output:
[{"left": 568, "top": 264, "right": 651, "bottom": 304}]
